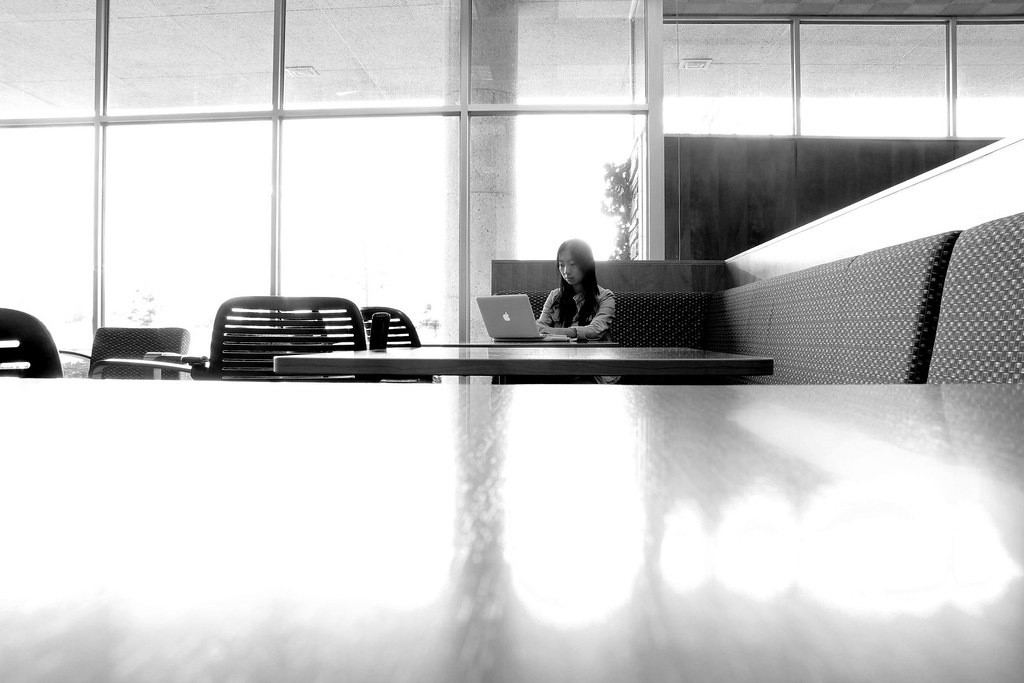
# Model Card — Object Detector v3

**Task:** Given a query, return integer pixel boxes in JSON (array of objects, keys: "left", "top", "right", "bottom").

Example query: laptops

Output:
[{"left": 475, "top": 294, "right": 554, "bottom": 339}]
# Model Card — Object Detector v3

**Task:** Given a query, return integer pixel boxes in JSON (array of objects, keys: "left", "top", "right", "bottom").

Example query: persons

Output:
[{"left": 535, "top": 239, "right": 621, "bottom": 384}]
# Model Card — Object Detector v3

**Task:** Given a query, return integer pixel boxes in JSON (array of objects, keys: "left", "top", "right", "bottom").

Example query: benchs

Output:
[{"left": 490, "top": 226, "right": 1022, "bottom": 384}]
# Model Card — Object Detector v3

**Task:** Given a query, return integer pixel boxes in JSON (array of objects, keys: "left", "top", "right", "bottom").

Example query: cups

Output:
[{"left": 369, "top": 312, "right": 391, "bottom": 351}]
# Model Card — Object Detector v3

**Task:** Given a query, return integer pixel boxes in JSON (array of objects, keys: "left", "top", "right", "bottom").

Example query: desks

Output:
[
  {"left": 420, "top": 340, "right": 618, "bottom": 347},
  {"left": 273, "top": 346, "right": 773, "bottom": 375}
]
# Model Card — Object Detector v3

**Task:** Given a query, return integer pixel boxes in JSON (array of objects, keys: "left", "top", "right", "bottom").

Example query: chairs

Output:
[{"left": 0, "top": 296, "right": 433, "bottom": 382}]
